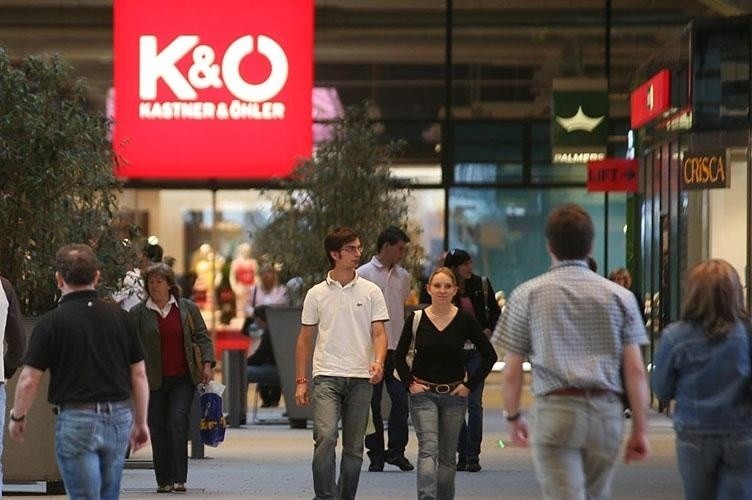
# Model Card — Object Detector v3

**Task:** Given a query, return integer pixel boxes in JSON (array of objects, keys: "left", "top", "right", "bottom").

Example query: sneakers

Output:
[
  {"left": 457, "top": 455, "right": 481, "bottom": 472},
  {"left": 157, "top": 483, "right": 186, "bottom": 492},
  {"left": 369, "top": 454, "right": 414, "bottom": 471}
]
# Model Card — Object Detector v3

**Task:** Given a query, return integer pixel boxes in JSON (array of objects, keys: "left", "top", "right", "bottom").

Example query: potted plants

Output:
[
  {"left": 0, "top": 45, "right": 151, "bottom": 496},
  {"left": 247, "top": 98, "right": 431, "bottom": 429}
]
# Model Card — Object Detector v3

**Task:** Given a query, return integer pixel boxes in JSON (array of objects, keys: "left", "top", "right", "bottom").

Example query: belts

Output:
[
  {"left": 60, "top": 399, "right": 128, "bottom": 411},
  {"left": 547, "top": 388, "right": 617, "bottom": 396},
  {"left": 412, "top": 377, "right": 462, "bottom": 394}
]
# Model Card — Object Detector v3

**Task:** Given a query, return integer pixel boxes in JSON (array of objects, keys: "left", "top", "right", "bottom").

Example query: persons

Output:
[
  {"left": 1, "top": 242, "right": 215, "bottom": 498},
  {"left": 420, "top": 248, "right": 503, "bottom": 472},
  {"left": 164, "top": 242, "right": 305, "bottom": 408},
  {"left": 296, "top": 227, "right": 391, "bottom": 499},
  {"left": 653, "top": 259, "right": 751, "bottom": 499},
  {"left": 392, "top": 267, "right": 499, "bottom": 499},
  {"left": 498, "top": 203, "right": 652, "bottom": 500},
  {"left": 356, "top": 225, "right": 414, "bottom": 472}
]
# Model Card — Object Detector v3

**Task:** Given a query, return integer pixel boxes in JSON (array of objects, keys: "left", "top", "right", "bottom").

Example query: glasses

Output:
[{"left": 341, "top": 248, "right": 363, "bottom": 252}]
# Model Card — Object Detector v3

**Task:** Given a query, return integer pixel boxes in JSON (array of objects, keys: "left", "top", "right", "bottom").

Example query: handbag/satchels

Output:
[
  {"left": 393, "top": 310, "right": 423, "bottom": 382},
  {"left": 198, "top": 381, "right": 226, "bottom": 446}
]
